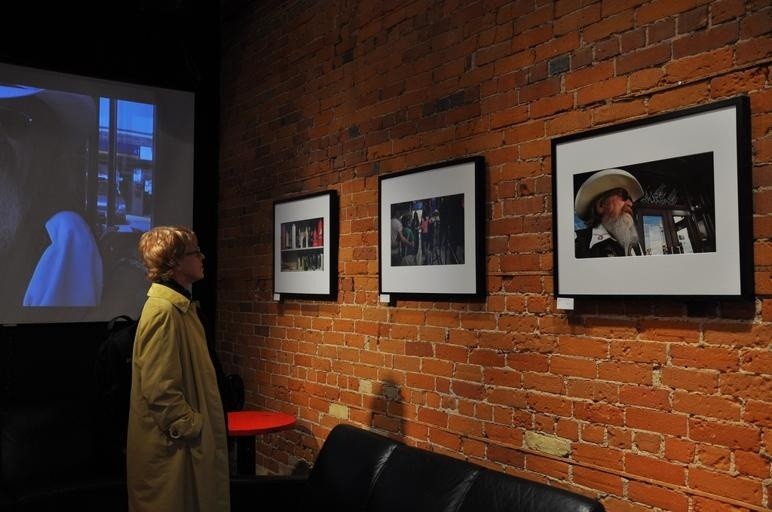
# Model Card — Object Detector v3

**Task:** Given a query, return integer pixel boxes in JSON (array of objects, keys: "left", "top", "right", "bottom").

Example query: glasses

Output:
[
  {"left": 181, "top": 249, "right": 200, "bottom": 259},
  {"left": 620, "top": 191, "right": 633, "bottom": 202}
]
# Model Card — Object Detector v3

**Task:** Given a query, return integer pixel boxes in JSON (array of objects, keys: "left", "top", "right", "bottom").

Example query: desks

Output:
[{"left": 226, "top": 409, "right": 296, "bottom": 474}]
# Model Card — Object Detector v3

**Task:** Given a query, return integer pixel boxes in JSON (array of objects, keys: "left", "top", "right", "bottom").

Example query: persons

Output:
[
  {"left": 125, "top": 227, "right": 232, "bottom": 510},
  {"left": 391, "top": 195, "right": 463, "bottom": 265},
  {"left": 573, "top": 167, "right": 644, "bottom": 257},
  {"left": 1, "top": 81, "right": 105, "bottom": 307}
]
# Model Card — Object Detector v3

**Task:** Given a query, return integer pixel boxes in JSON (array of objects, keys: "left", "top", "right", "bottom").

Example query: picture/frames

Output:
[
  {"left": 549, "top": 91, "right": 755, "bottom": 308},
  {"left": 272, "top": 187, "right": 341, "bottom": 303},
  {"left": 377, "top": 154, "right": 490, "bottom": 302}
]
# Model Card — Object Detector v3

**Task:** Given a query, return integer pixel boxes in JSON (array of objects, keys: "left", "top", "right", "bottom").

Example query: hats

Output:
[{"left": 575, "top": 168, "right": 645, "bottom": 221}]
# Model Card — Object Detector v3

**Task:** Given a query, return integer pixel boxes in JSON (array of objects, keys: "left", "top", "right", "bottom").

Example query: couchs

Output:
[{"left": 229, "top": 423, "right": 604, "bottom": 512}]
